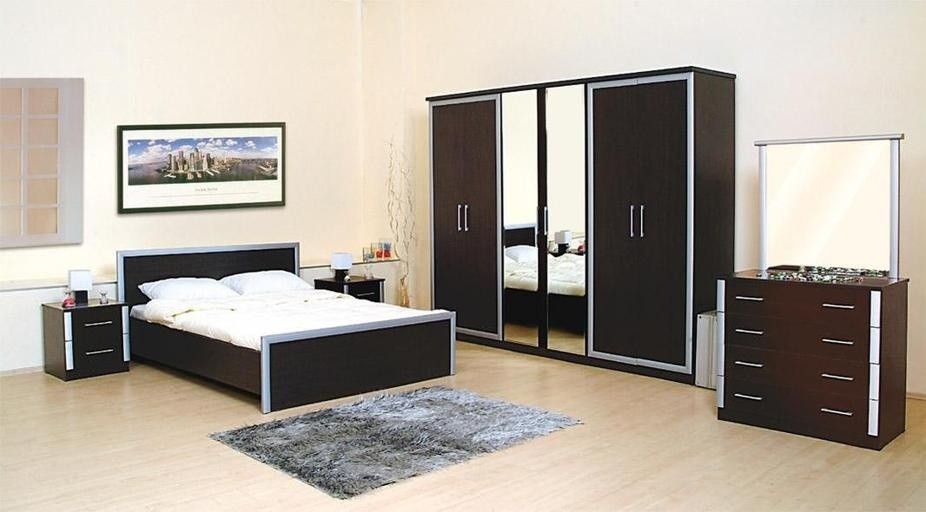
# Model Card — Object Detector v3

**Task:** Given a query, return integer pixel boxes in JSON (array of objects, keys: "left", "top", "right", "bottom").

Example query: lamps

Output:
[
  {"left": 68, "top": 270, "right": 92, "bottom": 303},
  {"left": 331, "top": 252, "right": 352, "bottom": 278}
]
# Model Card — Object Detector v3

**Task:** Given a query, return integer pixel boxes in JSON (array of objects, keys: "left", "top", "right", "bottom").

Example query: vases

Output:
[{"left": 399, "top": 286, "right": 409, "bottom": 308}]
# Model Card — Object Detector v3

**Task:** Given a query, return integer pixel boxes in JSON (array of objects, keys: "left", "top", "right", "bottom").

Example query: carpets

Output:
[{"left": 210, "top": 384, "right": 583, "bottom": 501}]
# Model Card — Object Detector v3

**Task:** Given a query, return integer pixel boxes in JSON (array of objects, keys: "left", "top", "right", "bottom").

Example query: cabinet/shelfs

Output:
[
  {"left": 423, "top": 66, "right": 735, "bottom": 385},
  {"left": 717, "top": 270, "right": 910, "bottom": 451}
]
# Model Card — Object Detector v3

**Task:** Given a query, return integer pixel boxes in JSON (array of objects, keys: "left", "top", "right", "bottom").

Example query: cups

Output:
[{"left": 361, "top": 236, "right": 392, "bottom": 263}]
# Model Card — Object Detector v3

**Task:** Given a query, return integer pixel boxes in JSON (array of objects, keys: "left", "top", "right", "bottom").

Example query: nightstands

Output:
[
  {"left": 314, "top": 275, "right": 385, "bottom": 304},
  {"left": 42, "top": 298, "right": 130, "bottom": 381}
]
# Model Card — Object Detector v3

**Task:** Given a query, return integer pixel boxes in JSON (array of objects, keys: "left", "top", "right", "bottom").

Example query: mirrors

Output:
[{"left": 501, "top": 84, "right": 587, "bottom": 360}]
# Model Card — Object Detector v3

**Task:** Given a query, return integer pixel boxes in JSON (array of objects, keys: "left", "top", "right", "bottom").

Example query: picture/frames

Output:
[{"left": 117, "top": 122, "right": 286, "bottom": 214}]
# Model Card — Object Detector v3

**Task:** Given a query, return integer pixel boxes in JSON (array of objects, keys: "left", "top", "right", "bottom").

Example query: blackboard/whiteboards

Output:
[{"left": 754, "top": 134, "right": 904, "bottom": 279}]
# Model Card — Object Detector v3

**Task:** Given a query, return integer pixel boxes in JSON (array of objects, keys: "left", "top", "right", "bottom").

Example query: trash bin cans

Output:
[{"left": 697, "top": 312, "right": 718, "bottom": 390}]
